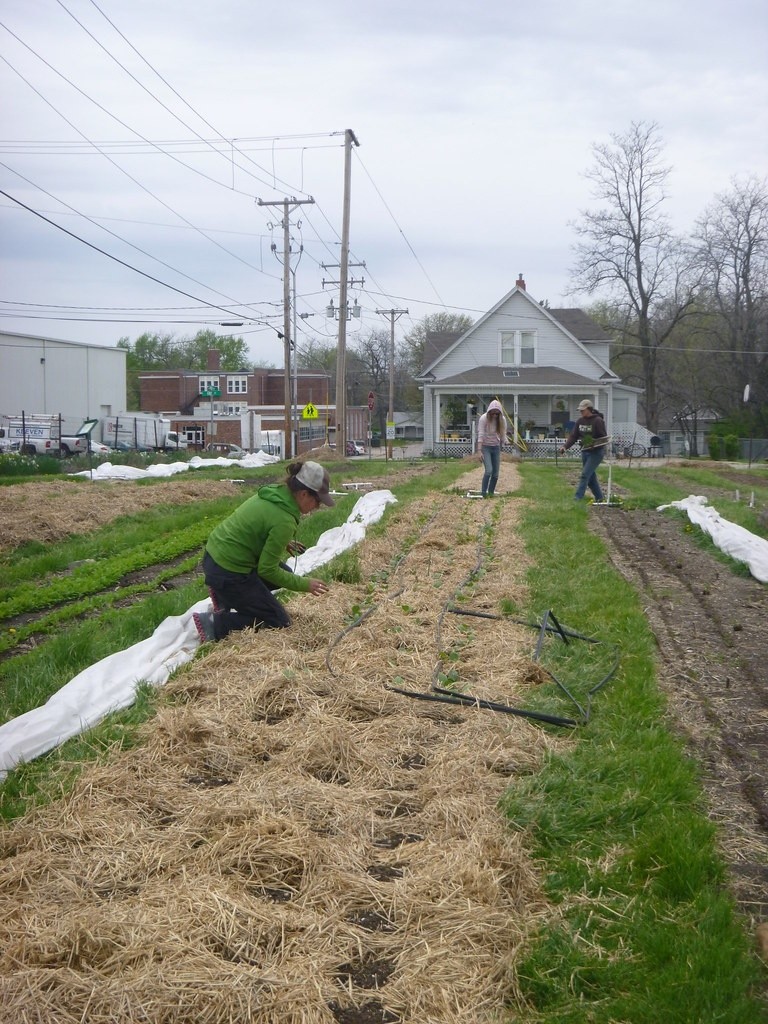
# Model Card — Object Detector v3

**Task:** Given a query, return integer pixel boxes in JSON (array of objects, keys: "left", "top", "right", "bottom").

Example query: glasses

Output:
[
  {"left": 305, "top": 487, "right": 321, "bottom": 502},
  {"left": 489, "top": 413, "right": 500, "bottom": 415}
]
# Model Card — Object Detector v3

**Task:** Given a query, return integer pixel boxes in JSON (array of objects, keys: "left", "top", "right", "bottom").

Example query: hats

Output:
[
  {"left": 292, "top": 461, "right": 336, "bottom": 507},
  {"left": 576, "top": 400, "right": 593, "bottom": 411}
]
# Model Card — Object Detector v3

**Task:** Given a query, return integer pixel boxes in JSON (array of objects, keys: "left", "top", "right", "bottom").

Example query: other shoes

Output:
[
  {"left": 209, "top": 587, "right": 230, "bottom": 615},
  {"left": 573, "top": 497, "right": 584, "bottom": 502},
  {"left": 193, "top": 612, "right": 218, "bottom": 645},
  {"left": 593, "top": 497, "right": 603, "bottom": 506}
]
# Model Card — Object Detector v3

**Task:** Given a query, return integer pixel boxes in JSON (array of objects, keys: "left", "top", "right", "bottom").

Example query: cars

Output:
[
  {"left": 311, "top": 443, "right": 336, "bottom": 450},
  {"left": 355, "top": 445, "right": 365, "bottom": 456},
  {"left": 85, "top": 439, "right": 110, "bottom": 455},
  {"left": 58, "top": 434, "right": 86, "bottom": 458},
  {"left": 200, "top": 443, "right": 245, "bottom": 453},
  {"left": 100, "top": 439, "right": 154, "bottom": 454}
]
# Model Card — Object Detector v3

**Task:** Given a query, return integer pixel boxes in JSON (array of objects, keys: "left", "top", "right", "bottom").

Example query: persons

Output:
[
  {"left": 193, "top": 461, "right": 336, "bottom": 645},
  {"left": 477, "top": 399, "right": 509, "bottom": 496},
  {"left": 559, "top": 400, "right": 608, "bottom": 502}
]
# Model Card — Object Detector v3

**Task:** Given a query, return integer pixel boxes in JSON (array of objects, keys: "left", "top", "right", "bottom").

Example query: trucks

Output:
[
  {"left": 0, "top": 409, "right": 66, "bottom": 456},
  {"left": 101, "top": 415, "right": 188, "bottom": 455}
]
